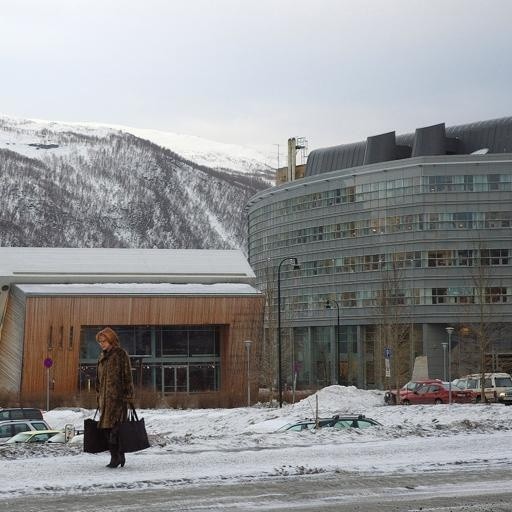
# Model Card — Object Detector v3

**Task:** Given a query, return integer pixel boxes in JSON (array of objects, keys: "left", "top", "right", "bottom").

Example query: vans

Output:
[{"left": 466, "top": 372, "right": 512, "bottom": 403}]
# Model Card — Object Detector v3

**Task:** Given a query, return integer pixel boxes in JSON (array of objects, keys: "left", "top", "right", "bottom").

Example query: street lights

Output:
[
  {"left": 277, "top": 257, "right": 301, "bottom": 408},
  {"left": 243, "top": 340, "right": 253, "bottom": 407},
  {"left": 326, "top": 298, "right": 340, "bottom": 385},
  {"left": 441, "top": 327, "right": 455, "bottom": 404}
]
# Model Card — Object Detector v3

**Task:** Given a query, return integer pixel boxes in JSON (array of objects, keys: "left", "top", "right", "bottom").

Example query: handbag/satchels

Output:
[
  {"left": 83, "top": 405, "right": 110, "bottom": 453},
  {"left": 117, "top": 400, "right": 151, "bottom": 454}
]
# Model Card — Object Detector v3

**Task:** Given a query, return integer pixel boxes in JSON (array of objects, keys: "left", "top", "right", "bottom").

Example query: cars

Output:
[
  {"left": 272, "top": 414, "right": 383, "bottom": 432},
  {"left": 0, "top": 407, "right": 84, "bottom": 444},
  {"left": 384, "top": 378, "right": 477, "bottom": 405}
]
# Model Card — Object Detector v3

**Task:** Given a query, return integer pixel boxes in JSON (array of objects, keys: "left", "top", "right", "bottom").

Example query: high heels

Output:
[{"left": 107, "top": 454, "right": 125, "bottom": 468}]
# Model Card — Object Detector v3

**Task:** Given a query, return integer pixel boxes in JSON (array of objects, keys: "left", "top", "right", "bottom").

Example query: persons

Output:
[{"left": 94, "top": 327, "right": 136, "bottom": 469}]
